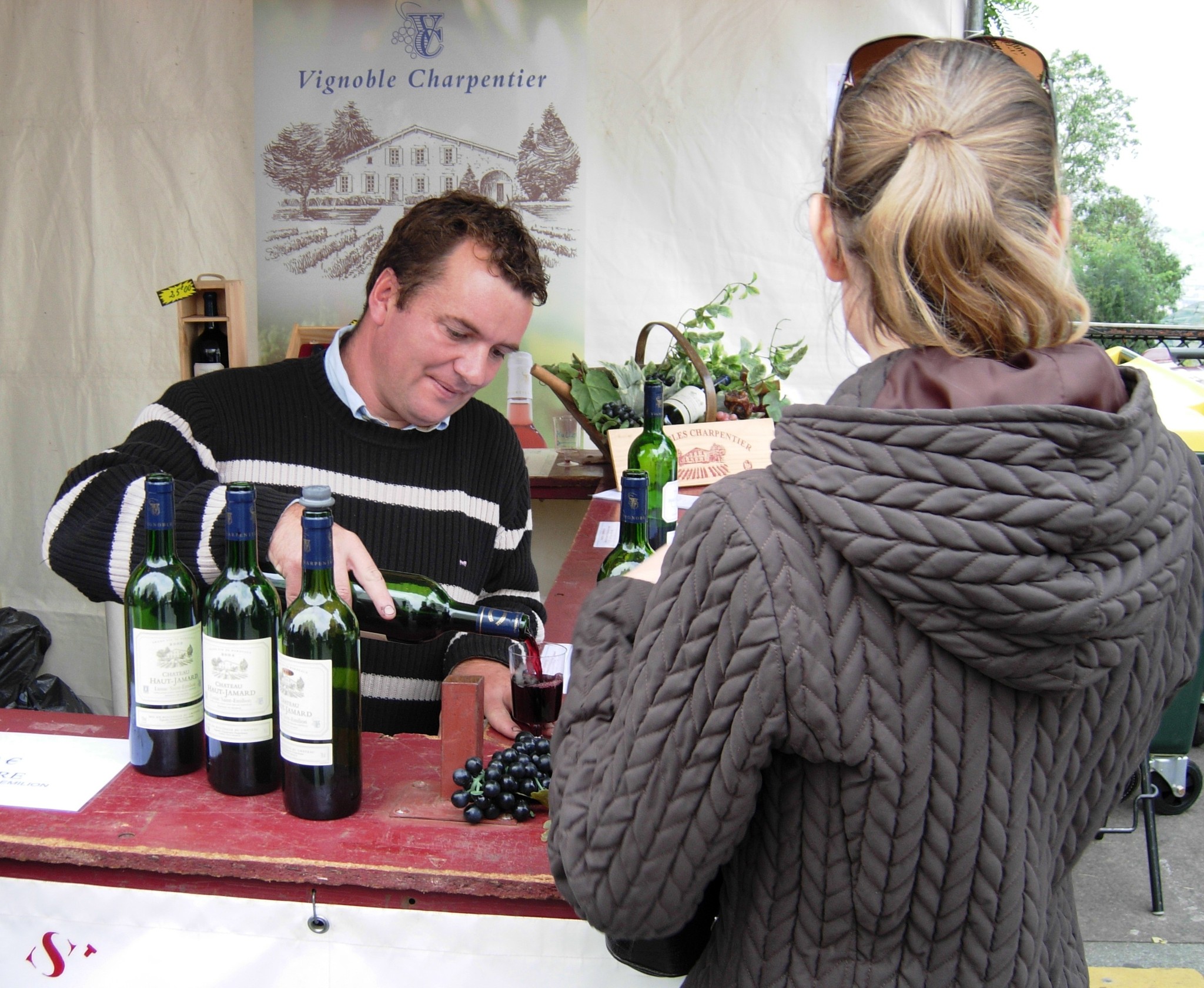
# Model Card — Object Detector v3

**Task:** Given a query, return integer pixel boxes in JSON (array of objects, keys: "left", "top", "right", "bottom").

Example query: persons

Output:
[
  {"left": 41, "top": 191, "right": 548, "bottom": 741},
  {"left": 548, "top": 34, "right": 1204, "bottom": 988}
]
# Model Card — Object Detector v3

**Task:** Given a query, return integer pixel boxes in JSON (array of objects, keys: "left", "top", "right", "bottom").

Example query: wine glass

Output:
[
  {"left": 552, "top": 415, "right": 582, "bottom": 467},
  {"left": 508, "top": 640, "right": 567, "bottom": 739}
]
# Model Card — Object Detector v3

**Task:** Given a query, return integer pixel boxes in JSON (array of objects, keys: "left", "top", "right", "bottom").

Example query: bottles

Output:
[
  {"left": 192, "top": 291, "right": 228, "bottom": 379},
  {"left": 278, "top": 483, "right": 363, "bottom": 821},
  {"left": 506, "top": 350, "right": 548, "bottom": 448},
  {"left": 124, "top": 471, "right": 204, "bottom": 779},
  {"left": 663, "top": 373, "right": 732, "bottom": 425},
  {"left": 202, "top": 480, "right": 282, "bottom": 797},
  {"left": 596, "top": 468, "right": 658, "bottom": 584},
  {"left": 256, "top": 560, "right": 532, "bottom": 648},
  {"left": 626, "top": 378, "right": 679, "bottom": 553}
]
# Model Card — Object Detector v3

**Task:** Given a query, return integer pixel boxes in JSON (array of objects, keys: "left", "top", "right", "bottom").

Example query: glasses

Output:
[{"left": 823, "top": 33, "right": 1059, "bottom": 198}]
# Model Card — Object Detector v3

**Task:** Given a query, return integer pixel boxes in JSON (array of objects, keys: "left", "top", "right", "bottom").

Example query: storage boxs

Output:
[
  {"left": 177, "top": 274, "right": 247, "bottom": 380},
  {"left": 285, "top": 324, "right": 342, "bottom": 359}
]
